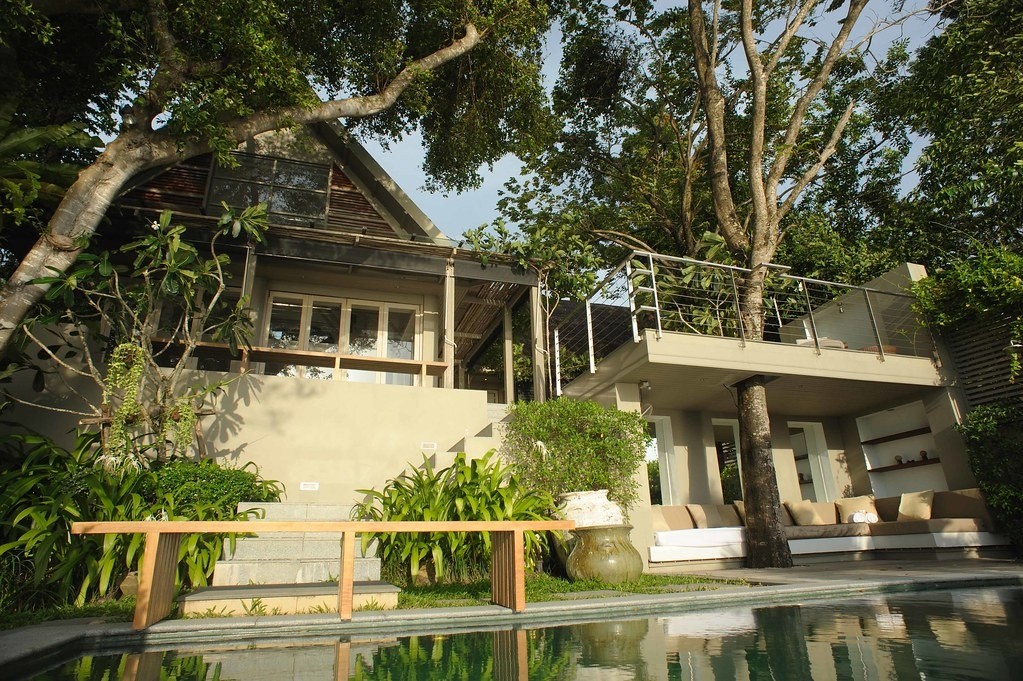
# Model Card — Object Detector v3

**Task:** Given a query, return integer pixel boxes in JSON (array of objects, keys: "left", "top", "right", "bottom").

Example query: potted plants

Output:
[
  {"left": 495, "top": 395, "right": 654, "bottom": 568},
  {"left": 350, "top": 448, "right": 571, "bottom": 587}
]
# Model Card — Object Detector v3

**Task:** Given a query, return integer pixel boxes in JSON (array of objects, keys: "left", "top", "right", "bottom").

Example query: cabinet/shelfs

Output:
[
  {"left": 861, "top": 426, "right": 941, "bottom": 472},
  {"left": 794, "top": 454, "right": 814, "bottom": 484}
]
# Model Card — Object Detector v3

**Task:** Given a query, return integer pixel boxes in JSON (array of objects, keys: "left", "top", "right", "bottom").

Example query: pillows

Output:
[
  {"left": 784, "top": 498, "right": 825, "bottom": 525},
  {"left": 733, "top": 500, "right": 746, "bottom": 526},
  {"left": 651, "top": 504, "right": 671, "bottom": 531},
  {"left": 687, "top": 503, "right": 724, "bottom": 528},
  {"left": 834, "top": 493, "right": 883, "bottom": 523},
  {"left": 897, "top": 488, "right": 934, "bottom": 521}
]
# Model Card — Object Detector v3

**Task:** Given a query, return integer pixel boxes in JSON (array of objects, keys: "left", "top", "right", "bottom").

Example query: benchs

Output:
[{"left": 71, "top": 521, "right": 577, "bottom": 631}]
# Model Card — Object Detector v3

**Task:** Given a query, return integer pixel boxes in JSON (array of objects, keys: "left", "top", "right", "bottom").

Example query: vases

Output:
[{"left": 566, "top": 523, "right": 643, "bottom": 582}]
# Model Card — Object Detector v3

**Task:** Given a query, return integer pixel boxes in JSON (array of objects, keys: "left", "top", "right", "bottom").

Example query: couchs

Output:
[{"left": 647, "top": 487, "right": 997, "bottom": 562}]
